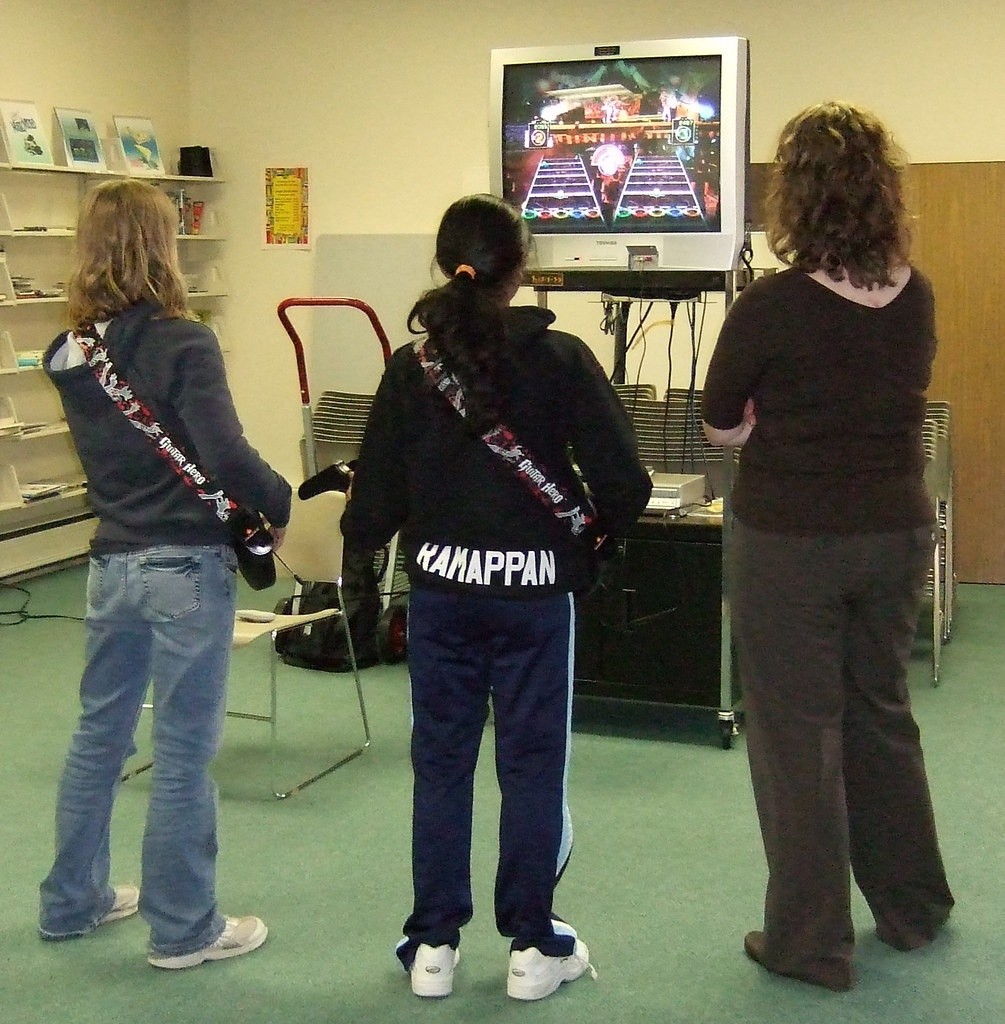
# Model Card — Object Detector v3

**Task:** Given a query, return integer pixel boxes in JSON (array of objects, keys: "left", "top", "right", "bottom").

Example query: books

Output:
[
  {"left": 0, "top": 418, "right": 45, "bottom": 437},
  {"left": 0, "top": 101, "right": 166, "bottom": 174},
  {"left": 165, "top": 188, "right": 204, "bottom": 235},
  {"left": 21, "top": 484, "right": 62, "bottom": 503},
  {"left": 17, "top": 350, "right": 45, "bottom": 366},
  {"left": 12, "top": 276, "right": 68, "bottom": 299}
]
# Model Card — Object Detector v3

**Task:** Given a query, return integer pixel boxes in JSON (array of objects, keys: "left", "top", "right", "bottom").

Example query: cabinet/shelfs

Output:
[
  {"left": 572, "top": 503, "right": 741, "bottom": 751},
  {"left": 0, "top": 163, "right": 229, "bottom": 585}
]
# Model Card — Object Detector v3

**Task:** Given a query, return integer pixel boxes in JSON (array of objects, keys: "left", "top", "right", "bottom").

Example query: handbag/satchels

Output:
[
  {"left": 233, "top": 506, "right": 276, "bottom": 590},
  {"left": 274, "top": 574, "right": 382, "bottom": 671}
]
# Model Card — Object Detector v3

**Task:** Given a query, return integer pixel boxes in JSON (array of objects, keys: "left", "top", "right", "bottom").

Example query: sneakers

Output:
[
  {"left": 409, "top": 943, "right": 460, "bottom": 997},
  {"left": 148, "top": 915, "right": 268, "bottom": 969},
  {"left": 101, "top": 883, "right": 139, "bottom": 923},
  {"left": 507, "top": 938, "right": 597, "bottom": 1001}
]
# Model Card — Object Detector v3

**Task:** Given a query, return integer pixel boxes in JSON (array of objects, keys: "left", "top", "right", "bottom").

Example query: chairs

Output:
[{"left": 118, "top": 488, "right": 371, "bottom": 798}]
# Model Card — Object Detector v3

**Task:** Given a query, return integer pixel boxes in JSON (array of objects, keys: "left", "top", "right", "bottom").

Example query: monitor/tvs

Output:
[{"left": 488, "top": 35, "right": 749, "bottom": 272}]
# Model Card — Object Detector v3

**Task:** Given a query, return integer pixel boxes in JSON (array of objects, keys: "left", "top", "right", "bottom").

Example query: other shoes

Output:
[{"left": 745, "top": 929, "right": 765, "bottom": 964}]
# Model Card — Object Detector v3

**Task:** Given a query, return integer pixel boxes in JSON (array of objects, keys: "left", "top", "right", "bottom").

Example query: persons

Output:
[
  {"left": 702, "top": 100, "right": 954, "bottom": 990},
  {"left": 38, "top": 180, "right": 292, "bottom": 971},
  {"left": 338, "top": 194, "right": 653, "bottom": 999}
]
